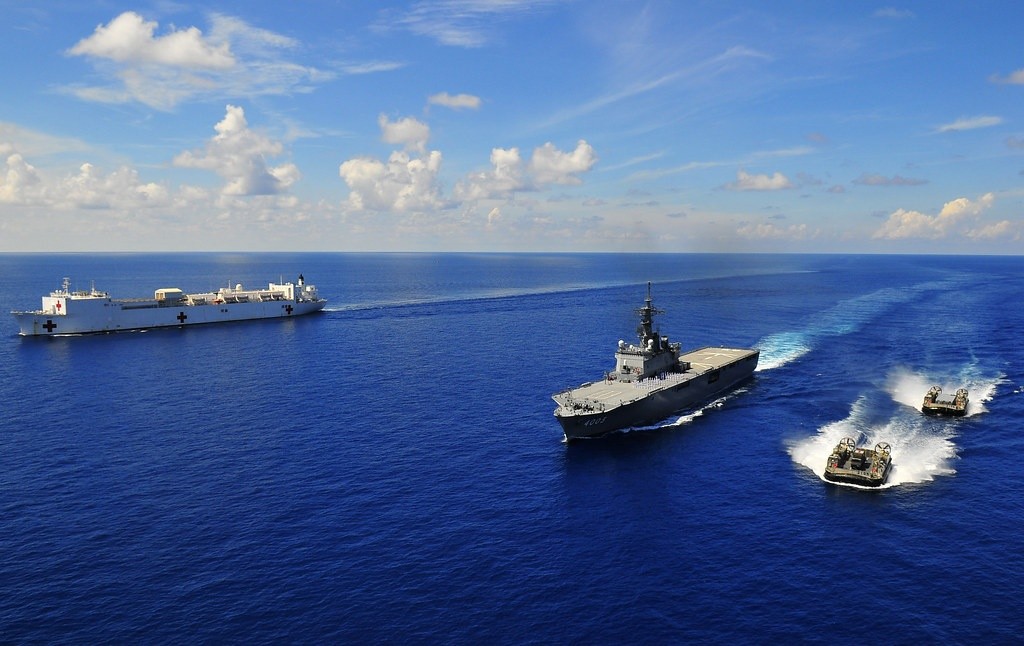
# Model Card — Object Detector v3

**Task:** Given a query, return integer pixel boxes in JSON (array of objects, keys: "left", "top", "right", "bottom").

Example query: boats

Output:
[
  {"left": 547, "top": 279, "right": 762, "bottom": 440},
  {"left": 922, "top": 386, "right": 970, "bottom": 416},
  {"left": 824, "top": 437, "right": 894, "bottom": 487},
  {"left": 7, "top": 274, "right": 327, "bottom": 336}
]
